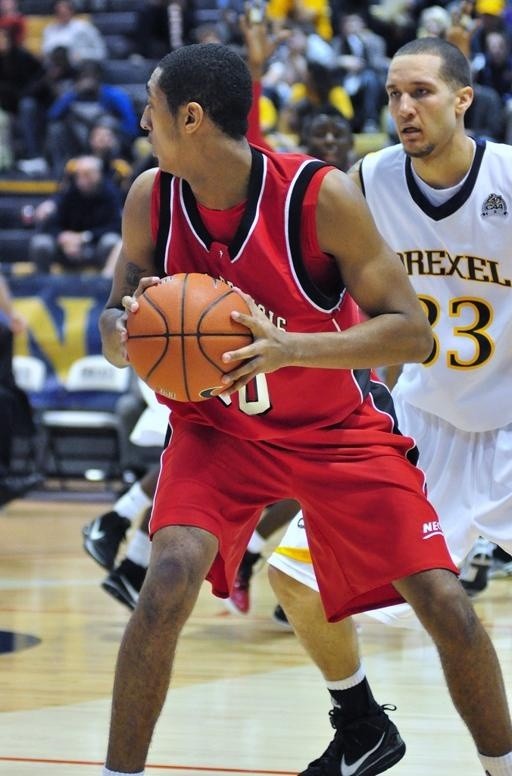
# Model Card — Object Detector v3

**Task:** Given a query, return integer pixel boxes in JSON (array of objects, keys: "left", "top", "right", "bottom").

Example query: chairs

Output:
[
  {"left": 12, "top": 354, "right": 48, "bottom": 497},
  {"left": 39, "top": 352, "right": 136, "bottom": 494}
]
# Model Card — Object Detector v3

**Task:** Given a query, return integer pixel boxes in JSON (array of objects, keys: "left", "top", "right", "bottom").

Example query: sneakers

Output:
[
  {"left": 227, "top": 548, "right": 264, "bottom": 616},
  {"left": 457, "top": 543, "right": 493, "bottom": 598},
  {"left": 271, "top": 605, "right": 289, "bottom": 627},
  {"left": 81, "top": 511, "right": 131, "bottom": 572},
  {"left": 98, "top": 558, "right": 149, "bottom": 613},
  {"left": 297, "top": 695, "right": 411, "bottom": 776}
]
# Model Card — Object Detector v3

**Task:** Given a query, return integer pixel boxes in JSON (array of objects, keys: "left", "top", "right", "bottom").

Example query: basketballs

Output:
[{"left": 126, "top": 273, "right": 253, "bottom": 405}]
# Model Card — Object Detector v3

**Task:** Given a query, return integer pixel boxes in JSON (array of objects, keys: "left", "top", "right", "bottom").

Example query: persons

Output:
[
  {"left": 101, "top": 45, "right": 512, "bottom": 776},
  {"left": 266, "top": 37, "right": 512, "bottom": 776}
]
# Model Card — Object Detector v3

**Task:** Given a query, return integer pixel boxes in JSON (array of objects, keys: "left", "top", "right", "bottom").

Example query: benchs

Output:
[{"left": 1, "top": 0, "right": 511, "bottom": 296}]
